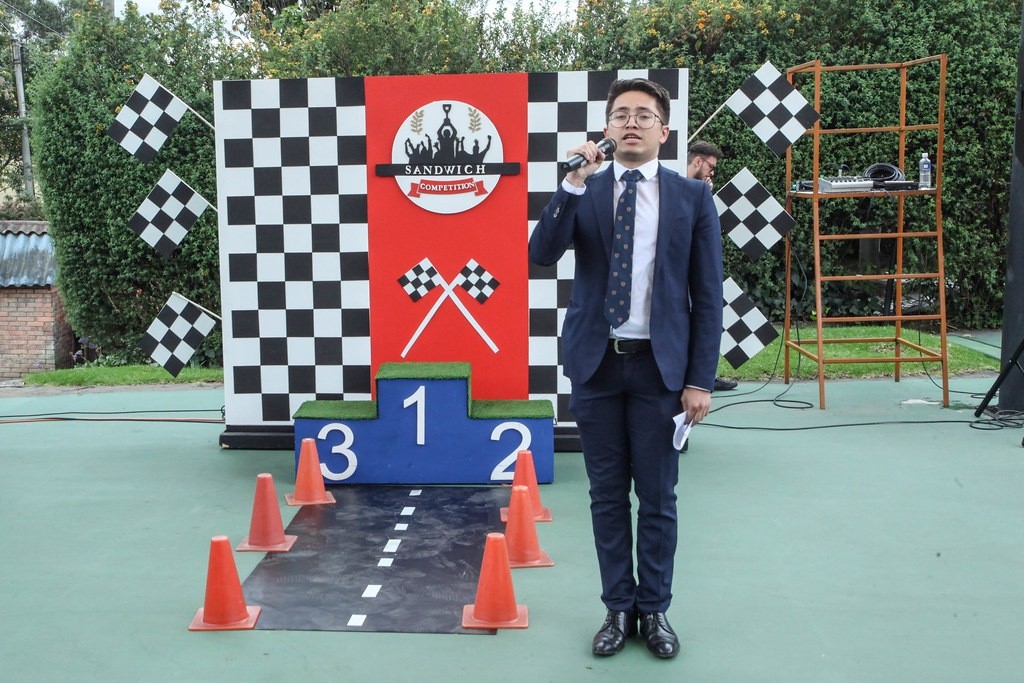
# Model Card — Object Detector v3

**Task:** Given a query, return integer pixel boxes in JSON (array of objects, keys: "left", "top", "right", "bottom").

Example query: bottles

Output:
[{"left": 919, "top": 152, "right": 932, "bottom": 190}]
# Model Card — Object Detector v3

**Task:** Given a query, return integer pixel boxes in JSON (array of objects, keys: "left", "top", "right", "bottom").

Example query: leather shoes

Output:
[
  {"left": 592, "top": 609, "right": 638, "bottom": 656},
  {"left": 639, "top": 612, "right": 681, "bottom": 658}
]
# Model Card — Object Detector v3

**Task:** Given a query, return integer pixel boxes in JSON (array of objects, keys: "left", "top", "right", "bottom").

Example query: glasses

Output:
[
  {"left": 608, "top": 110, "right": 665, "bottom": 130},
  {"left": 701, "top": 157, "right": 716, "bottom": 171}
]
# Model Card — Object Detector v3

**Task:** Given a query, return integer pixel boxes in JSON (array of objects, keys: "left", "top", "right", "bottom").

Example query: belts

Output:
[{"left": 607, "top": 339, "right": 651, "bottom": 355}]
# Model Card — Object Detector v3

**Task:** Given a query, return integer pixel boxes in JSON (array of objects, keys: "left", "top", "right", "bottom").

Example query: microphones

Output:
[{"left": 561, "top": 138, "right": 617, "bottom": 173}]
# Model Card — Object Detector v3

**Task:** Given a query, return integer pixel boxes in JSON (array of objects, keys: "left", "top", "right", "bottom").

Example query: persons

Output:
[
  {"left": 687, "top": 141, "right": 738, "bottom": 390},
  {"left": 528, "top": 79, "right": 723, "bottom": 658}
]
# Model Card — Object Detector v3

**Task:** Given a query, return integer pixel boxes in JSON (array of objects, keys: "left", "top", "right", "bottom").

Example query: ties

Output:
[{"left": 602, "top": 170, "right": 644, "bottom": 329}]
